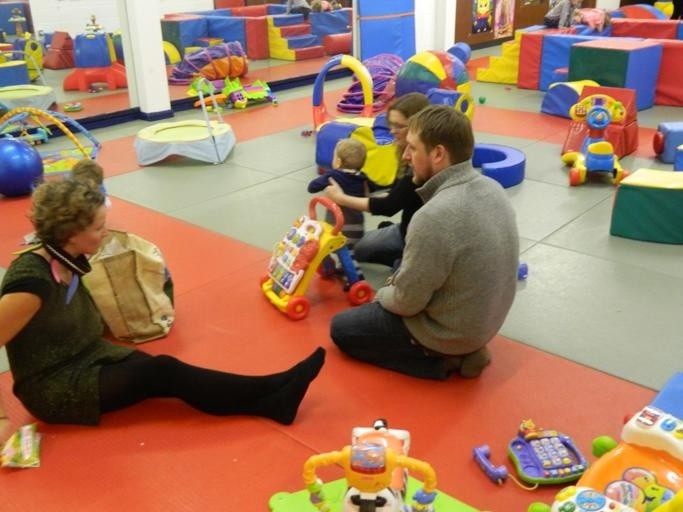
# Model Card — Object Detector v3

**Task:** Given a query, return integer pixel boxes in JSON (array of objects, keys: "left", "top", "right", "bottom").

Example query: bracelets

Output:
[{"left": 1, "top": 415, "right": 9, "bottom": 420}]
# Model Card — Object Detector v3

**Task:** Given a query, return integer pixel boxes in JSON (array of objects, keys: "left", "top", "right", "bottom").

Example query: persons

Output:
[
  {"left": 331, "top": 104, "right": 520, "bottom": 382},
  {"left": 0, "top": 177, "right": 326, "bottom": 445}
]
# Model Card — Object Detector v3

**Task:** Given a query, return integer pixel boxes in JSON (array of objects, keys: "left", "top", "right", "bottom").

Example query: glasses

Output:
[{"left": 385, "top": 119, "right": 408, "bottom": 131}]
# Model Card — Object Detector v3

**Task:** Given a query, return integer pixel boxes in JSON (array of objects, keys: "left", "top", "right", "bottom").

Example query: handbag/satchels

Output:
[{"left": 79, "top": 229, "right": 174, "bottom": 343}]
